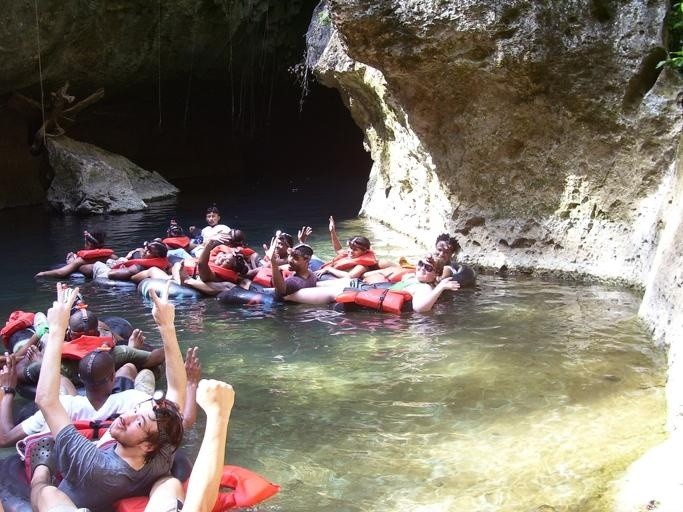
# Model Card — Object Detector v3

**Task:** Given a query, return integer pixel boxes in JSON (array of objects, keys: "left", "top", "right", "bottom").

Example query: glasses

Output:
[{"left": 417, "top": 259, "right": 436, "bottom": 272}]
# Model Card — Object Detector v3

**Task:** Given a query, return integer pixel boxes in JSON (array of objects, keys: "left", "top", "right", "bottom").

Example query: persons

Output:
[
  {"left": 0, "top": 279, "right": 237, "bottom": 512},
  {"left": 31, "top": 206, "right": 477, "bottom": 316}
]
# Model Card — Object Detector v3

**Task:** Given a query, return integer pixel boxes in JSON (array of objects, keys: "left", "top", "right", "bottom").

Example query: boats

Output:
[
  {"left": 90, "top": 259, "right": 477, "bottom": 306},
  {"left": 0, "top": 317, "right": 192, "bottom": 512}
]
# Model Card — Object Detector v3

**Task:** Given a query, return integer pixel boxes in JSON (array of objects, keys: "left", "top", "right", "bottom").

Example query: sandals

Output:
[{"left": 29, "top": 436, "right": 55, "bottom": 486}]
